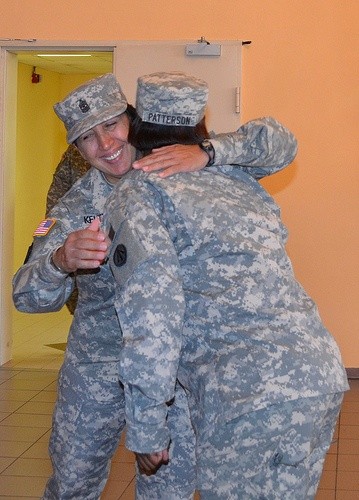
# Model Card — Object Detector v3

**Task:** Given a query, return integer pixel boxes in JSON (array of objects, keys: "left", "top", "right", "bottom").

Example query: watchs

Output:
[{"left": 200, "top": 139, "right": 215, "bottom": 168}]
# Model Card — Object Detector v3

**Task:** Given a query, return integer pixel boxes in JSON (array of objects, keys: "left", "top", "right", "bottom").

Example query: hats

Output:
[
  {"left": 135, "top": 73, "right": 210, "bottom": 126},
  {"left": 54, "top": 73, "right": 129, "bottom": 144}
]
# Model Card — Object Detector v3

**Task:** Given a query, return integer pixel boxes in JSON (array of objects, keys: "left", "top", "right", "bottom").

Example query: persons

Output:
[
  {"left": 45, "top": 138, "right": 90, "bottom": 316},
  {"left": 11, "top": 71, "right": 299, "bottom": 499},
  {"left": 101, "top": 69, "right": 350, "bottom": 500}
]
